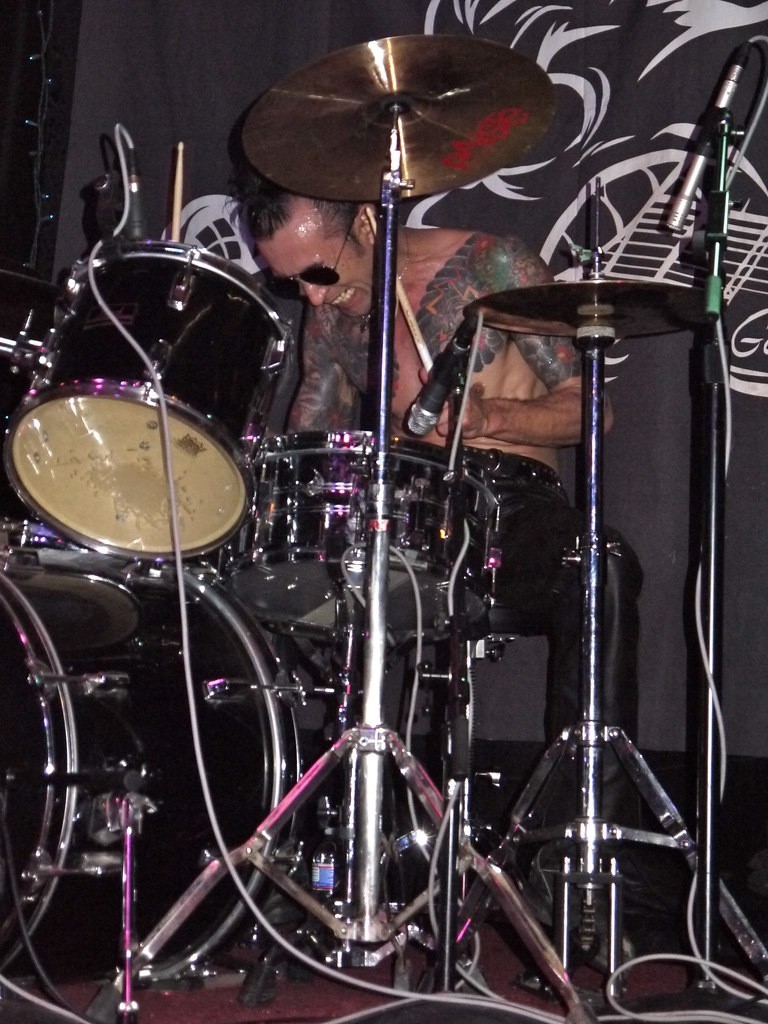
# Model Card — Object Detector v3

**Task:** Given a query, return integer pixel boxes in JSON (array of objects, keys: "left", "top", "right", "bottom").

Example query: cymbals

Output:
[
  {"left": 237, "top": 32, "right": 559, "bottom": 203},
  {"left": 461, "top": 278, "right": 728, "bottom": 340}
]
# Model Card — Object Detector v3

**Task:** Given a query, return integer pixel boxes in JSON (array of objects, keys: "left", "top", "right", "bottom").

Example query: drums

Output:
[
  {"left": 4, "top": 238, "right": 299, "bottom": 562},
  {"left": 1, "top": 555, "right": 303, "bottom": 985},
  {"left": 226, "top": 426, "right": 499, "bottom": 643}
]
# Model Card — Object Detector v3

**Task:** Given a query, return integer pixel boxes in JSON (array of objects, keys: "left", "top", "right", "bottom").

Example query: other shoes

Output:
[{"left": 529, "top": 840, "right": 693, "bottom": 974}]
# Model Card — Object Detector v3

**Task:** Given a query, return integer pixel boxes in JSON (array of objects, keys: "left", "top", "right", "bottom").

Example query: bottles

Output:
[{"left": 310, "top": 828, "right": 344, "bottom": 948}]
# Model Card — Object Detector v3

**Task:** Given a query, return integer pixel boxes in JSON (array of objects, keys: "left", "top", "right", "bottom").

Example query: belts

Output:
[{"left": 462, "top": 447, "right": 562, "bottom": 491}]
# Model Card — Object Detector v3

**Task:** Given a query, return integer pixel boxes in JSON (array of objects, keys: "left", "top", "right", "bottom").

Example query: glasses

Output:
[{"left": 263, "top": 218, "right": 354, "bottom": 300}]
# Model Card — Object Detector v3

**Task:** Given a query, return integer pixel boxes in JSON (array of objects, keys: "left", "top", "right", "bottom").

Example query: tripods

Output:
[{"left": 83, "top": 99, "right": 768, "bottom": 1023}]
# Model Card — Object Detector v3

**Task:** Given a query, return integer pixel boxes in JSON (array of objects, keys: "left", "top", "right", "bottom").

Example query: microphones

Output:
[
  {"left": 124, "top": 147, "right": 146, "bottom": 241},
  {"left": 404, "top": 322, "right": 477, "bottom": 436},
  {"left": 666, "top": 40, "right": 756, "bottom": 231}
]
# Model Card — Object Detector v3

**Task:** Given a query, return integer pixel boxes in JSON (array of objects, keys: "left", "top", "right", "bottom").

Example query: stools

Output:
[{"left": 431, "top": 588, "right": 560, "bottom": 799}]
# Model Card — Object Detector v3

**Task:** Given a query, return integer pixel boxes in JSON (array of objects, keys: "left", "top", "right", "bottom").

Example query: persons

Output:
[{"left": 229, "top": 174, "right": 681, "bottom": 925}]
